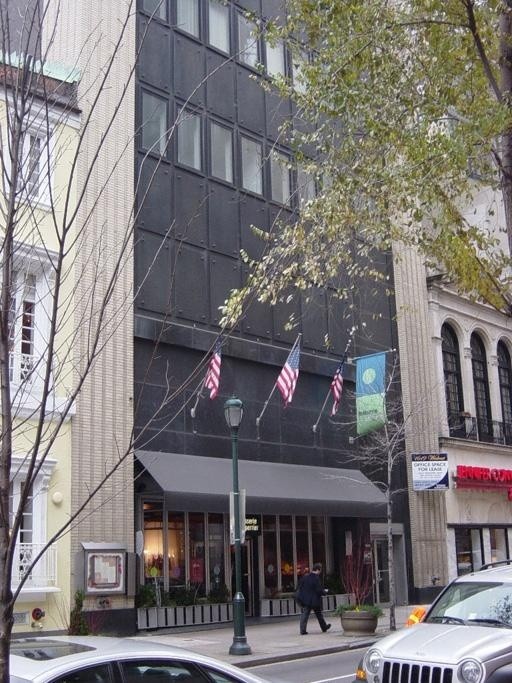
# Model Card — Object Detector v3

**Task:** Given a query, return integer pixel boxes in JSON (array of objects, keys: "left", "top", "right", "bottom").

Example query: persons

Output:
[{"left": 292, "top": 562, "right": 332, "bottom": 636}]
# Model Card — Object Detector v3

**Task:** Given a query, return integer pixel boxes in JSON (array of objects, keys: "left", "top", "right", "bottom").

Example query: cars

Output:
[{"left": 8, "top": 635, "right": 270, "bottom": 682}]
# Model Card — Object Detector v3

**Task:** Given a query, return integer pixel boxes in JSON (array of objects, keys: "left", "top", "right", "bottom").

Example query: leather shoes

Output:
[
  {"left": 301, "top": 631, "right": 307, "bottom": 634},
  {"left": 323, "top": 623, "right": 330, "bottom": 631}
]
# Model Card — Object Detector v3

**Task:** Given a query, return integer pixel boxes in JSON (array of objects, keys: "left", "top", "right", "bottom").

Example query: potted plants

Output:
[{"left": 333, "top": 603, "right": 385, "bottom": 636}]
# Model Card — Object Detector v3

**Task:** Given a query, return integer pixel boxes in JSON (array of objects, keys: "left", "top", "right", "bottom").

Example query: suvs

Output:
[{"left": 353, "top": 560, "right": 511, "bottom": 682}]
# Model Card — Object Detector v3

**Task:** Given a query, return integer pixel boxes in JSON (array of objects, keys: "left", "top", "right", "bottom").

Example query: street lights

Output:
[{"left": 222, "top": 392, "right": 251, "bottom": 654}]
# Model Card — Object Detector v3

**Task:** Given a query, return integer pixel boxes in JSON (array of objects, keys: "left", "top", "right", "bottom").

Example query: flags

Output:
[
  {"left": 328, "top": 342, "right": 353, "bottom": 419},
  {"left": 205, "top": 331, "right": 223, "bottom": 400},
  {"left": 275, "top": 336, "right": 301, "bottom": 410}
]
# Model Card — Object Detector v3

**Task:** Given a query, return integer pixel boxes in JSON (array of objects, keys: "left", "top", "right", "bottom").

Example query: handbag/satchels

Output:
[{"left": 292, "top": 582, "right": 307, "bottom": 608}]
